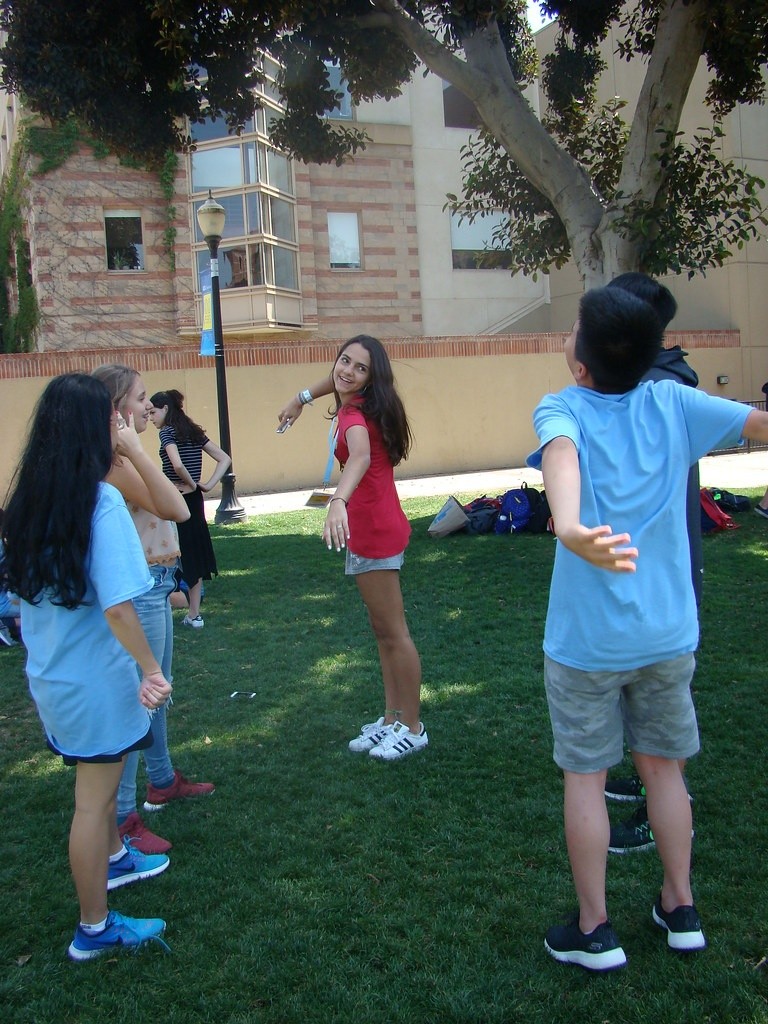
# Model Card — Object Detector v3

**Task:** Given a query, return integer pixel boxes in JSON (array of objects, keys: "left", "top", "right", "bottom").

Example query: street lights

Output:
[{"left": 196, "top": 188, "right": 248, "bottom": 525}]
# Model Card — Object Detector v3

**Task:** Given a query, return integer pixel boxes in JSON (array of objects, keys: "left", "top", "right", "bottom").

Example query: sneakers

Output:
[
  {"left": 180, "top": 615, "right": 204, "bottom": 628},
  {"left": 69, "top": 910, "right": 171, "bottom": 958},
  {"left": 119, "top": 812, "right": 173, "bottom": 854},
  {"left": 108, "top": 834, "right": 170, "bottom": 891},
  {"left": 144, "top": 770, "right": 215, "bottom": 811},
  {"left": 545, "top": 918, "right": 626, "bottom": 969},
  {"left": 0, "top": 619, "right": 19, "bottom": 646},
  {"left": 653, "top": 892, "right": 705, "bottom": 948},
  {"left": 609, "top": 800, "right": 694, "bottom": 852},
  {"left": 348, "top": 717, "right": 394, "bottom": 752},
  {"left": 604, "top": 775, "right": 692, "bottom": 800},
  {"left": 369, "top": 721, "right": 429, "bottom": 760}
]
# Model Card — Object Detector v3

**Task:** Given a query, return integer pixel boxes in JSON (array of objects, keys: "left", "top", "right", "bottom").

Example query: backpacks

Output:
[
  {"left": 709, "top": 487, "right": 750, "bottom": 513},
  {"left": 468, "top": 481, "right": 554, "bottom": 535}
]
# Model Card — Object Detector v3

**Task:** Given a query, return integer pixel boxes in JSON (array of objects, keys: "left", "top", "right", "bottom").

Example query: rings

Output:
[{"left": 337, "top": 526, "right": 342, "bottom": 529}]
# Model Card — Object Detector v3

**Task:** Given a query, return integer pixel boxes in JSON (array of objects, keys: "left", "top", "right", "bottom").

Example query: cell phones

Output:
[{"left": 276, "top": 421, "right": 289, "bottom": 433}]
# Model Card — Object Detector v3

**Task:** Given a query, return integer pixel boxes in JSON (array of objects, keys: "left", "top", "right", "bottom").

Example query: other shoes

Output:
[{"left": 754, "top": 503, "right": 768, "bottom": 519}]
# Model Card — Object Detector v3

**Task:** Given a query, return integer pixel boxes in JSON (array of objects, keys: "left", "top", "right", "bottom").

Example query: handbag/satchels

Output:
[{"left": 428, "top": 496, "right": 472, "bottom": 539}]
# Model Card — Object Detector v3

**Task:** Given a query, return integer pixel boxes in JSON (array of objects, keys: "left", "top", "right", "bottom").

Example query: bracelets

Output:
[
  {"left": 297, "top": 388, "right": 314, "bottom": 407},
  {"left": 330, "top": 497, "right": 346, "bottom": 505}
]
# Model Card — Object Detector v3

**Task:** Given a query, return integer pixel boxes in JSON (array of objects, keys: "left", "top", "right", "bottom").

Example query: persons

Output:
[
  {"left": 94, "top": 365, "right": 217, "bottom": 857},
  {"left": 0, "top": 507, "right": 20, "bottom": 646},
  {"left": 147, "top": 389, "right": 232, "bottom": 627},
  {"left": 527, "top": 283, "right": 768, "bottom": 973},
  {"left": 169, "top": 575, "right": 205, "bottom": 609},
  {"left": 0, "top": 374, "right": 173, "bottom": 962},
  {"left": 601, "top": 273, "right": 700, "bottom": 853},
  {"left": 754, "top": 382, "right": 768, "bottom": 519},
  {"left": 277, "top": 335, "right": 428, "bottom": 759}
]
